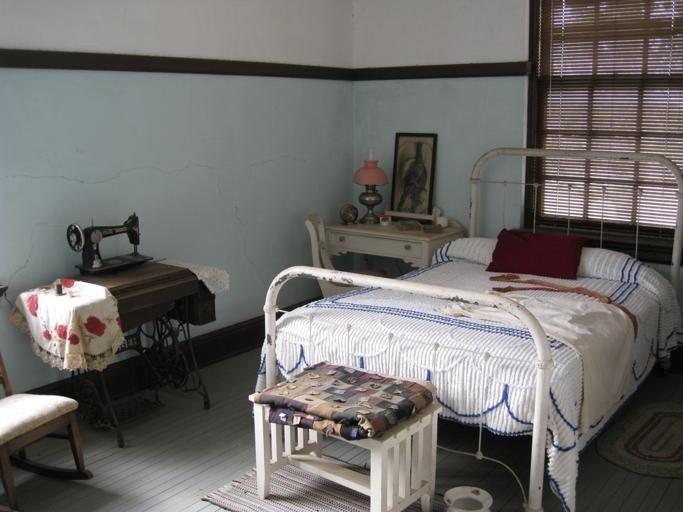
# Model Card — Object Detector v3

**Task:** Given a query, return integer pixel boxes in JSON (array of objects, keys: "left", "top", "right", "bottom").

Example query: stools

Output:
[{"left": 249, "top": 390, "right": 443, "bottom": 511}]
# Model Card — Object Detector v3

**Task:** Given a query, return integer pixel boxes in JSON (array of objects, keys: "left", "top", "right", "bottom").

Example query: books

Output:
[
  {"left": 395, "top": 218, "right": 423, "bottom": 231},
  {"left": 423, "top": 224, "right": 444, "bottom": 235}
]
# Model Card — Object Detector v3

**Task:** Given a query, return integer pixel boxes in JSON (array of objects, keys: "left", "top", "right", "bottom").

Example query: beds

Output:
[{"left": 261, "top": 146, "right": 682, "bottom": 511}]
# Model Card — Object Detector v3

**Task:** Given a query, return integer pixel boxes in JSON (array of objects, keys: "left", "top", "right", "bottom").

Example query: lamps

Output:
[{"left": 351, "top": 159, "right": 389, "bottom": 224}]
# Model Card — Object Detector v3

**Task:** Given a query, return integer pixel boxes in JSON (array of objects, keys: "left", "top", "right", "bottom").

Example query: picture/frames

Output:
[{"left": 390, "top": 132, "right": 438, "bottom": 226}]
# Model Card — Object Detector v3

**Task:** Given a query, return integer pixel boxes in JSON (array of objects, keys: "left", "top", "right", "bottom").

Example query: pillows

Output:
[{"left": 485, "top": 228, "right": 586, "bottom": 281}]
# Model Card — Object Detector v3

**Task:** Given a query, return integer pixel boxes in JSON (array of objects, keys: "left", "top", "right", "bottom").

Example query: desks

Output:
[{"left": 321, "top": 223, "right": 465, "bottom": 269}]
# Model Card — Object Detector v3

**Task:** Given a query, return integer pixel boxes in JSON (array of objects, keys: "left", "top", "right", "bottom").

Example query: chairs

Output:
[
  {"left": 0, "top": 356, "right": 93, "bottom": 511},
  {"left": 302, "top": 213, "right": 362, "bottom": 298}
]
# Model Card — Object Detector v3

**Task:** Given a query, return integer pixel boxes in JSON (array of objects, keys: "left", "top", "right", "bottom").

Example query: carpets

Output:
[
  {"left": 199, "top": 453, "right": 450, "bottom": 511},
  {"left": 594, "top": 400, "right": 683, "bottom": 480}
]
